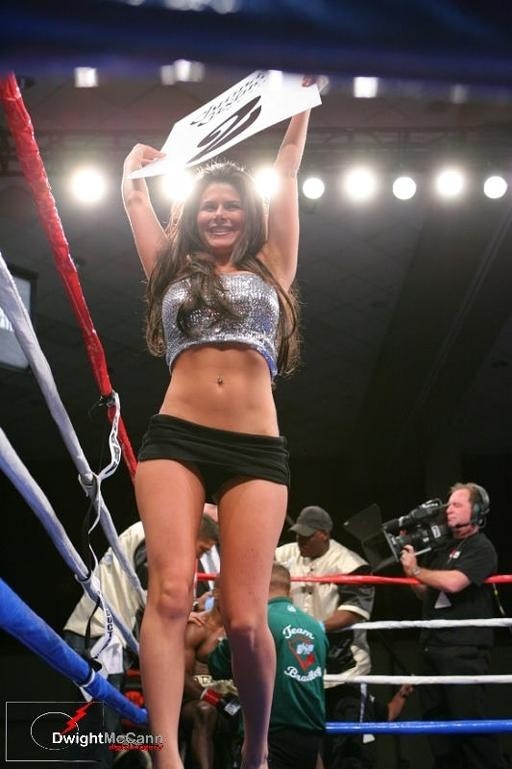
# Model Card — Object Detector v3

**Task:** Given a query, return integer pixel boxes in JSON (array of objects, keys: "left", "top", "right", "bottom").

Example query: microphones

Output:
[{"left": 456, "top": 519, "right": 477, "bottom": 528}]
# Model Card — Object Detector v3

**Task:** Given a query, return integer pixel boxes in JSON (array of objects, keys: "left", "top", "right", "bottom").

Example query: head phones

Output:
[{"left": 468, "top": 482, "right": 490, "bottom": 528}]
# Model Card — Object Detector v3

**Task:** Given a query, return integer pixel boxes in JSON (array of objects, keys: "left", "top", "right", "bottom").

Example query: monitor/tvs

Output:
[{"left": 0, "top": 261, "right": 39, "bottom": 375}]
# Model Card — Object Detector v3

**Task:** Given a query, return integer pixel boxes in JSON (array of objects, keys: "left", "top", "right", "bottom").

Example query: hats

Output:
[{"left": 288, "top": 505, "right": 334, "bottom": 537}]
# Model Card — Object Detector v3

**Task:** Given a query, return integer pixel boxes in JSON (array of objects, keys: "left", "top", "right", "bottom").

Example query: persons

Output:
[
  {"left": 120, "top": 76, "right": 328, "bottom": 769},
  {"left": 399, "top": 482, "right": 506, "bottom": 769}
]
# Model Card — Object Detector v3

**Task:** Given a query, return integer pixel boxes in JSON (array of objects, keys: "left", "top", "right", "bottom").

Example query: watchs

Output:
[{"left": 411, "top": 566, "right": 421, "bottom": 576}]
